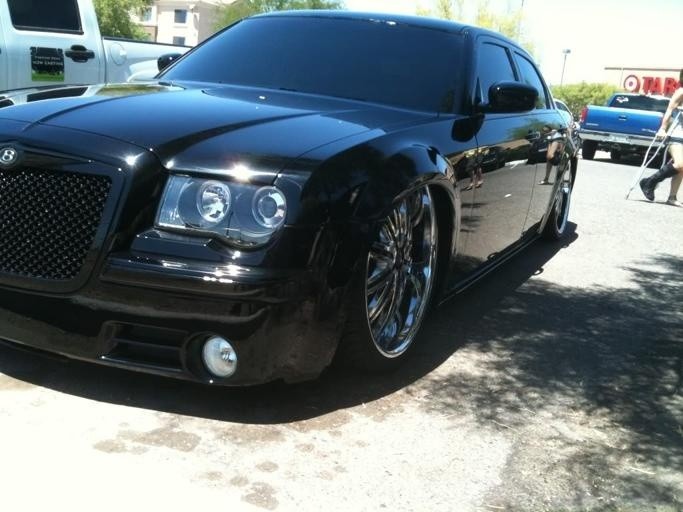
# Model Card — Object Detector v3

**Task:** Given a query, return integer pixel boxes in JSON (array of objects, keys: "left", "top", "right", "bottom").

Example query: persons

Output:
[
  {"left": 537, "top": 125, "right": 565, "bottom": 184},
  {"left": 473, "top": 145, "right": 490, "bottom": 190},
  {"left": 465, "top": 148, "right": 478, "bottom": 189},
  {"left": 638, "top": 68, "right": 683, "bottom": 210}
]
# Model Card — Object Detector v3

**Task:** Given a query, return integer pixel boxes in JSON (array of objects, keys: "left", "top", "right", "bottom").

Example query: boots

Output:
[{"left": 640, "top": 163, "right": 679, "bottom": 201}]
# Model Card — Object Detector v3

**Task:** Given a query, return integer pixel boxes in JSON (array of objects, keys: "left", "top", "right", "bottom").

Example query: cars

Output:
[
  {"left": 1, "top": 9, "right": 578, "bottom": 391},
  {"left": 553, "top": 98, "right": 576, "bottom": 130}
]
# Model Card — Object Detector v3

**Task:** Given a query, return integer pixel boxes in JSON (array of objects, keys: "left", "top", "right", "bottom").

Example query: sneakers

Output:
[{"left": 666, "top": 199, "right": 683, "bottom": 207}]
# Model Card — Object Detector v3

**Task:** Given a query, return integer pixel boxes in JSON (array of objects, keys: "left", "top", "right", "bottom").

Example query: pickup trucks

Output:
[
  {"left": 0, "top": 0, "right": 193, "bottom": 93},
  {"left": 577, "top": 93, "right": 673, "bottom": 169}
]
